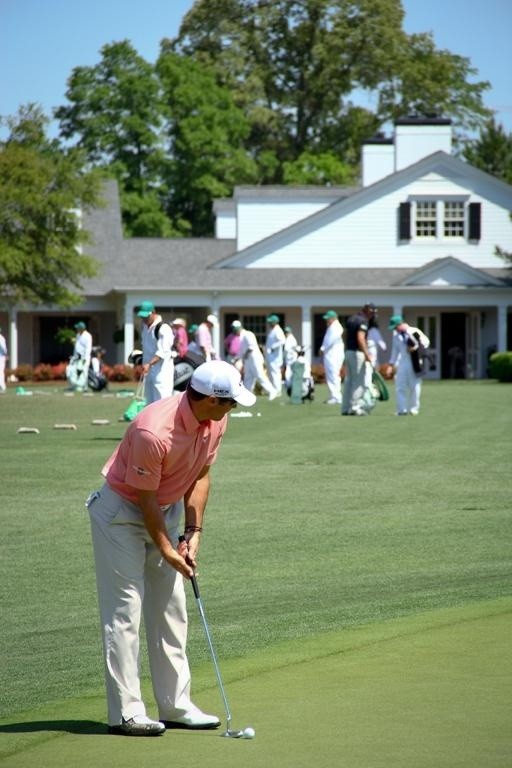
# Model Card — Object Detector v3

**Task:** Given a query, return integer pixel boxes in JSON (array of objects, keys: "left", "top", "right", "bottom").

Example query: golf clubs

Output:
[{"left": 178, "top": 536, "right": 243, "bottom": 737}]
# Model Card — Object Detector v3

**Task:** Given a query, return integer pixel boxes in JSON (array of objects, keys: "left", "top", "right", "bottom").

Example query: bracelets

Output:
[{"left": 185, "top": 526, "right": 201, "bottom": 533}]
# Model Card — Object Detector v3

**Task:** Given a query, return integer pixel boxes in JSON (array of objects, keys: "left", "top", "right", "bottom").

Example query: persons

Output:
[
  {"left": 223, "top": 333, "right": 240, "bottom": 363},
  {"left": 230, "top": 320, "right": 278, "bottom": 400},
  {"left": 283, "top": 325, "right": 297, "bottom": 380},
  {"left": 366, "top": 319, "right": 387, "bottom": 368},
  {"left": 136, "top": 300, "right": 219, "bottom": 405},
  {"left": 261, "top": 314, "right": 286, "bottom": 397},
  {"left": 64, "top": 320, "right": 94, "bottom": 394},
  {"left": 318, "top": 309, "right": 345, "bottom": 404},
  {"left": 384, "top": 315, "right": 430, "bottom": 416},
  {"left": 341, "top": 303, "right": 377, "bottom": 416},
  {"left": 0, "top": 328, "right": 9, "bottom": 394},
  {"left": 85, "top": 359, "right": 257, "bottom": 737}
]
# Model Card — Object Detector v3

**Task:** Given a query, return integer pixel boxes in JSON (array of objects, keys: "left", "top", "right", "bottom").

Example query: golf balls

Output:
[{"left": 243, "top": 728, "right": 255, "bottom": 738}]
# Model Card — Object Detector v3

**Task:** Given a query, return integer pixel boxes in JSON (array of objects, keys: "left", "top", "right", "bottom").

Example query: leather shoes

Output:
[
  {"left": 109, "top": 715, "right": 166, "bottom": 736},
  {"left": 159, "top": 709, "right": 220, "bottom": 729}
]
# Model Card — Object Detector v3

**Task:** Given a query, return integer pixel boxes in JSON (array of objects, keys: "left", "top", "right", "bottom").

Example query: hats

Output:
[
  {"left": 188, "top": 325, "right": 199, "bottom": 333},
  {"left": 190, "top": 359, "right": 259, "bottom": 407},
  {"left": 172, "top": 318, "right": 185, "bottom": 326},
  {"left": 387, "top": 316, "right": 404, "bottom": 331},
  {"left": 136, "top": 301, "right": 155, "bottom": 318},
  {"left": 266, "top": 315, "right": 279, "bottom": 323},
  {"left": 323, "top": 310, "right": 337, "bottom": 320},
  {"left": 231, "top": 320, "right": 241, "bottom": 335},
  {"left": 74, "top": 321, "right": 86, "bottom": 329},
  {"left": 207, "top": 315, "right": 219, "bottom": 328}
]
